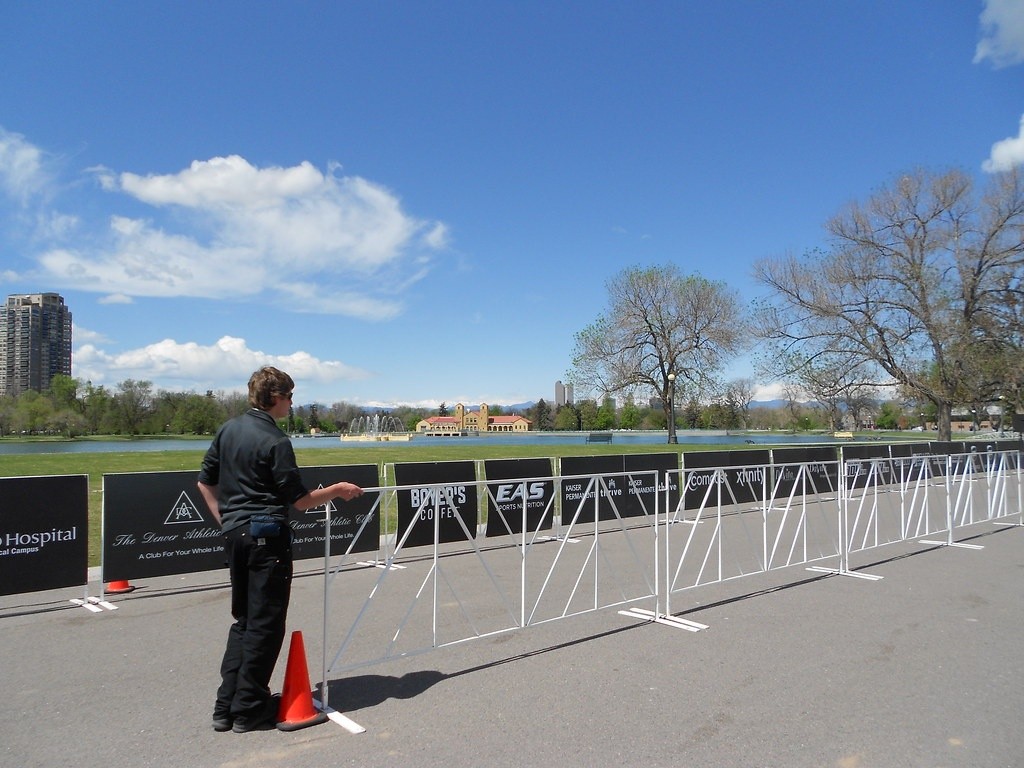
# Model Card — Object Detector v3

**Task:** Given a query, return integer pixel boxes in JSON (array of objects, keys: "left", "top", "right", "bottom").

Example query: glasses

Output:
[{"left": 280, "top": 392, "right": 293, "bottom": 401}]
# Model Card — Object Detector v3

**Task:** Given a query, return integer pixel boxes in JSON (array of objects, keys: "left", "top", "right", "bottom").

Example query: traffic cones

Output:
[
  {"left": 103, "top": 580, "right": 136, "bottom": 593},
  {"left": 266, "top": 629, "right": 329, "bottom": 732}
]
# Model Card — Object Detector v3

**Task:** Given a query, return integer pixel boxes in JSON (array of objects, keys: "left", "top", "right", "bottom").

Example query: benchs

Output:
[{"left": 586, "top": 433, "right": 612, "bottom": 444}]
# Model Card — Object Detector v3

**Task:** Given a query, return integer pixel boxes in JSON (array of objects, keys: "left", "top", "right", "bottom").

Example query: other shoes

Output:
[
  {"left": 232, "top": 694, "right": 280, "bottom": 732},
  {"left": 211, "top": 713, "right": 232, "bottom": 732}
]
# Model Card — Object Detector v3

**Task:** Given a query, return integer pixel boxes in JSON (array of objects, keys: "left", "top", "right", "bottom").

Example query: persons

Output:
[{"left": 199, "top": 368, "right": 363, "bottom": 732}]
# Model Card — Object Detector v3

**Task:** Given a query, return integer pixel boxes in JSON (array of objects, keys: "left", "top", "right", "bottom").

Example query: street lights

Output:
[
  {"left": 997, "top": 396, "right": 1006, "bottom": 439},
  {"left": 667, "top": 373, "right": 680, "bottom": 444}
]
